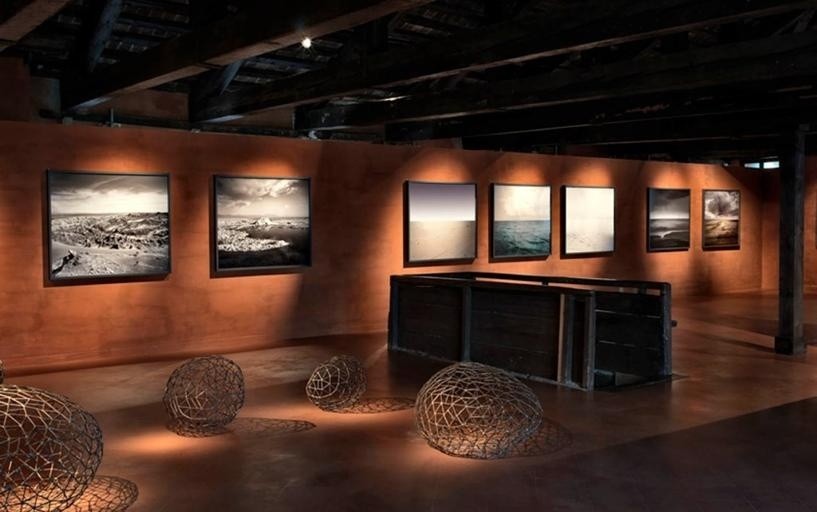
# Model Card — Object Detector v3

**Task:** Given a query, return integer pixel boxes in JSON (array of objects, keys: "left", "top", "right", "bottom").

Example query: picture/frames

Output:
[
  {"left": 702, "top": 188, "right": 741, "bottom": 250},
  {"left": 402, "top": 179, "right": 478, "bottom": 267},
  {"left": 646, "top": 186, "right": 691, "bottom": 252},
  {"left": 489, "top": 182, "right": 552, "bottom": 263},
  {"left": 559, "top": 184, "right": 617, "bottom": 260},
  {"left": 41, "top": 166, "right": 315, "bottom": 289}
]
